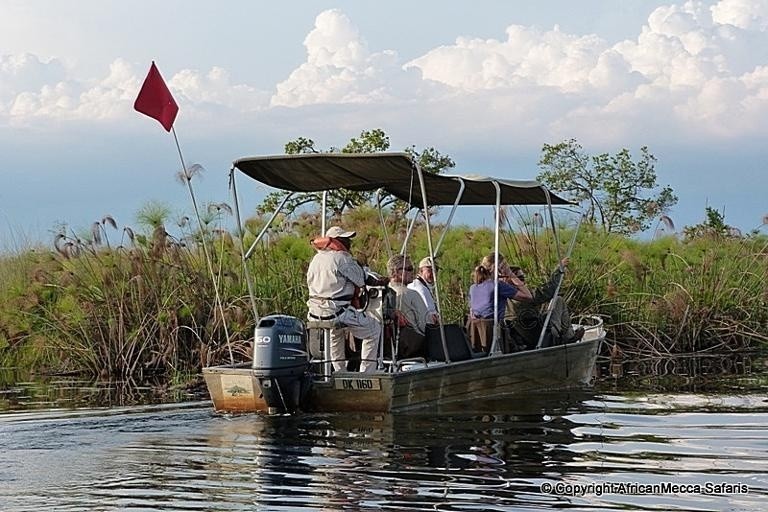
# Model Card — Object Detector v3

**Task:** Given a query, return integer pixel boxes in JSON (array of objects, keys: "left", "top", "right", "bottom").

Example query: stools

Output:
[{"left": 306, "top": 319, "right": 345, "bottom": 382}]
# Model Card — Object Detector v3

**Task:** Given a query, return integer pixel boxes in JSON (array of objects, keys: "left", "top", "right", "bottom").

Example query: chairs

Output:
[{"left": 424, "top": 320, "right": 488, "bottom": 361}]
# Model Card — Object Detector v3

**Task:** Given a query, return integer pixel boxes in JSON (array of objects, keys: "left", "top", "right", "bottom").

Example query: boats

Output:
[{"left": 201, "top": 327, "right": 607, "bottom": 417}]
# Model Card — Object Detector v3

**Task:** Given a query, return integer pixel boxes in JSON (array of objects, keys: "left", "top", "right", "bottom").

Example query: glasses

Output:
[{"left": 397, "top": 265, "right": 413, "bottom": 272}]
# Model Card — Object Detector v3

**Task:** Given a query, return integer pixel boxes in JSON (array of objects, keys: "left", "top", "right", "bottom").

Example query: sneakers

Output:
[{"left": 570, "top": 326, "right": 584, "bottom": 343}]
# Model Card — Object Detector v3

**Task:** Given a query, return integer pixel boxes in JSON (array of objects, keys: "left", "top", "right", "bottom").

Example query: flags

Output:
[{"left": 132, "top": 60, "right": 179, "bottom": 134}]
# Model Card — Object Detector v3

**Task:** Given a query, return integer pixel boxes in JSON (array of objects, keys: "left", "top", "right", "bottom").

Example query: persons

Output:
[
  {"left": 366, "top": 251, "right": 431, "bottom": 337},
  {"left": 304, "top": 223, "right": 390, "bottom": 374},
  {"left": 407, "top": 255, "right": 442, "bottom": 324},
  {"left": 468, "top": 251, "right": 534, "bottom": 321},
  {"left": 505, "top": 256, "right": 587, "bottom": 349}
]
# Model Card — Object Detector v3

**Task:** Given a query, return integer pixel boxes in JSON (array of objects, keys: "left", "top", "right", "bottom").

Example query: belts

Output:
[{"left": 308, "top": 304, "right": 348, "bottom": 321}]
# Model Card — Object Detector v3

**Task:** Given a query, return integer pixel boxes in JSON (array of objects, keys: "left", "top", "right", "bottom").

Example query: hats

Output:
[
  {"left": 419, "top": 257, "right": 442, "bottom": 271},
  {"left": 324, "top": 226, "right": 356, "bottom": 239}
]
